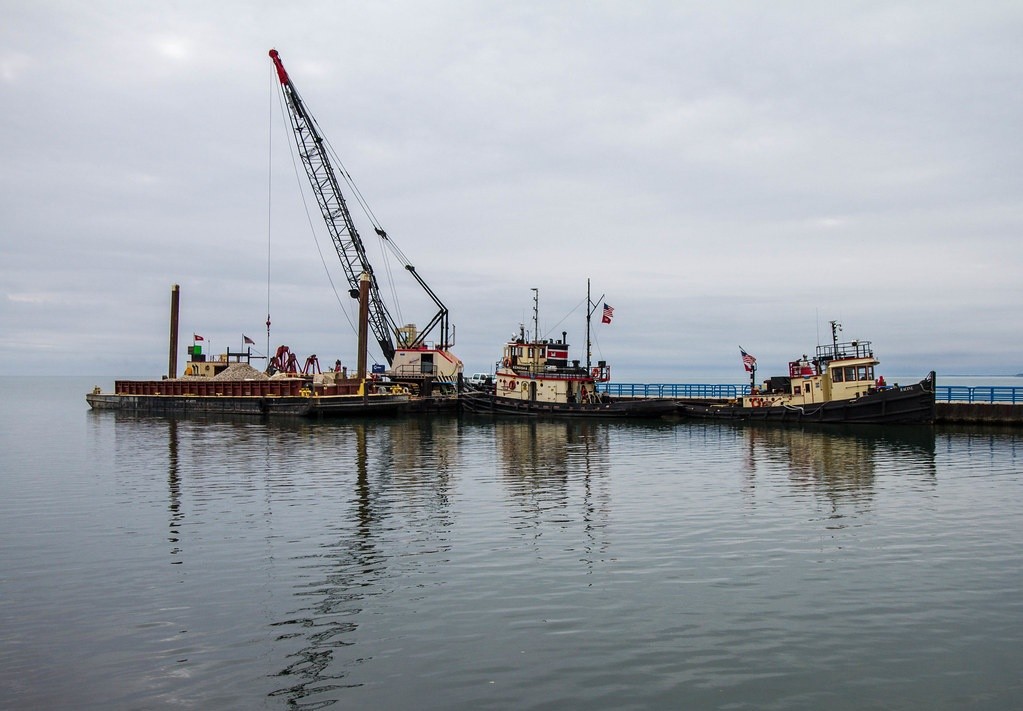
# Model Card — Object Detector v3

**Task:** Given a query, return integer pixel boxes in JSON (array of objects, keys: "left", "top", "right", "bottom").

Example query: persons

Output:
[
  {"left": 336, "top": 359, "right": 341, "bottom": 372},
  {"left": 876, "top": 376, "right": 886, "bottom": 386},
  {"left": 799, "top": 355, "right": 812, "bottom": 374},
  {"left": 812, "top": 357, "right": 822, "bottom": 374},
  {"left": 864, "top": 373, "right": 871, "bottom": 380}
]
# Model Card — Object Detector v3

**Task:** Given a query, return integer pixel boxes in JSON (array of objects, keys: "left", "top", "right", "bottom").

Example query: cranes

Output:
[{"left": 265, "top": 47, "right": 465, "bottom": 396}]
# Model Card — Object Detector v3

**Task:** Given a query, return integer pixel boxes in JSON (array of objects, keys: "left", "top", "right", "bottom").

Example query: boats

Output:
[
  {"left": 676, "top": 319, "right": 936, "bottom": 426},
  {"left": 466, "top": 278, "right": 674, "bottom": 420}
]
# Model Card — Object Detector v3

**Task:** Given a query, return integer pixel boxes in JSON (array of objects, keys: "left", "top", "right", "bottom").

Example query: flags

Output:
[
  {"left": 603, "top": 303, "right": 614, "bottom": 317},
  {"left": 244, "top": 335, "right": 255, "bottom": 345},
  {"left": 195, "top": 335, "right": 203, "bottom": 341},
  {"left": 602, "top": 315, "right": 611, "bottom": 324},
  {"left": 744, "top": 364, "right": 752, "bottom": 372},
  {"left": 741, "top": 350, "right": 756, "bottom": 365}
]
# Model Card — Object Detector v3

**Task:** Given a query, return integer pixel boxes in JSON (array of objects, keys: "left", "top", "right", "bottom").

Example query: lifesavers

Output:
[
  {"left": 592, "top": 369, "right": 599, "bottom": 378},
  {"left": 752, "top": 397, "right": 761, "bottom": 408}
]
